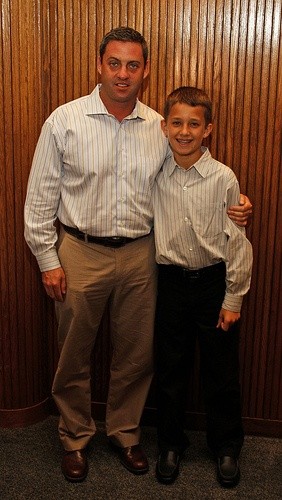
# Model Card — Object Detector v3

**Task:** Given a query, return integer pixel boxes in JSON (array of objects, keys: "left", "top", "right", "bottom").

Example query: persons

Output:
[
  {"left": 23, "top": 26, "right": 253, "bottom": 482},
  {"left": 152, "top": 86, "right": 254, "bottom": 488}
]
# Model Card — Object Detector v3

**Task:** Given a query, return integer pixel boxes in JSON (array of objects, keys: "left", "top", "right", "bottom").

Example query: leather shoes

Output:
[
  {"left": 119, "top": 444, "right": 148, "bottom": 475},
  {"left": 216, "top": 454, "right": 240, "bottom": 488},
  {"left": 156, "top": 449, "right": 180, "bottom": 484},
  {"left": 61, "top": 449, "right": 87, "bottom": 483}
]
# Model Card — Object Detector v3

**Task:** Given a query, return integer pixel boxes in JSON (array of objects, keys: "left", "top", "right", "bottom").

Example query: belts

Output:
[
  {"left": 63, "top": 224, "right": 154, "bottom": 249},
  {"left": 158, "top": 261, "right": 226, "bottom": 280}
]
